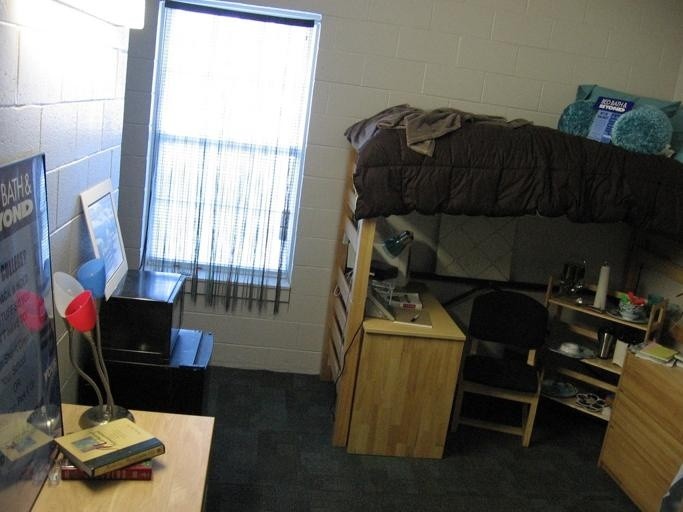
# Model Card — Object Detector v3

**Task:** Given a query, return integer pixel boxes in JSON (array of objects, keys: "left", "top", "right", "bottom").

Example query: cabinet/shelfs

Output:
[
  {"left": 75, "top": 330, "right": 215, "bottom": 416},
  {"left": 545, "top": 272, "right": 667, "bottom": 421}
]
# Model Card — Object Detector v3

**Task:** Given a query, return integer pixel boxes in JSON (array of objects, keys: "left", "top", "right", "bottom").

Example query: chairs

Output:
[{"left": 451, "top": 291, "right": 549, "bottom": 449}]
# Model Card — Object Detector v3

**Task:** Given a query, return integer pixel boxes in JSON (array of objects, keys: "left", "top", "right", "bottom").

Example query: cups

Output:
[{"left": 619, "top": 301, "right": 644, "bottom": 321}]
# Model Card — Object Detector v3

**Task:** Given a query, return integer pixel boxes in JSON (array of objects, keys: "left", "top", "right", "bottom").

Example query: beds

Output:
[{"left": 318, "top": 89, "right": 682, "bottom": 446}]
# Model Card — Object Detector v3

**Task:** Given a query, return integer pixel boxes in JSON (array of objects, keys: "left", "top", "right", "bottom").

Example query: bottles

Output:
[{"left": 596, "top": 325, "right": 615, "bottom": 361}]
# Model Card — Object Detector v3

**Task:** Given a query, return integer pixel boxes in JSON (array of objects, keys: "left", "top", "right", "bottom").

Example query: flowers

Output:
[{"left": 618, "top": 290, "right": 646, "bottom": 306}]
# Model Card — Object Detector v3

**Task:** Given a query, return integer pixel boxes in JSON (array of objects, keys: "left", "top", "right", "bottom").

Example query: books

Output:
[
  {"left": 630, "top": 341, "right": 682, "bottom": 369},
  {"left": 60, "top": 454, "right": 151, "bottom": 481},
  {"left": 54, "top": 417, "right": 164, "bottom": 477},
  {"left": 379, "top": 291, "right": 422, "bottom": 310},
  {"left": 0, "top": 420, "right": 55, "bottom": 467}
]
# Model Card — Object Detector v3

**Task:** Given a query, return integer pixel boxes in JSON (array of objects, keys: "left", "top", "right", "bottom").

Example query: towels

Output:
[{"left": 341, "top": 101, "right": 535, "bottom": 155}]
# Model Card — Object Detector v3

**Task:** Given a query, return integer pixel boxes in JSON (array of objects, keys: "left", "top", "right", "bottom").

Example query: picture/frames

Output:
[{"left": 79, "top": 177, "right": 126, "bottom": 302}]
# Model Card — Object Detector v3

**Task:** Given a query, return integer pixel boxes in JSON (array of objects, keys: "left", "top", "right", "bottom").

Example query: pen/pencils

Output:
[{"left": 411, "top": 312, "right": 420, "bottom": 322}]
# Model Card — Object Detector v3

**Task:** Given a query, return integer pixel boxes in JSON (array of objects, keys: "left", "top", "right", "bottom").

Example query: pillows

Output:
[{"left": 559, "top": 83, "right": 683, "bottom": 159}]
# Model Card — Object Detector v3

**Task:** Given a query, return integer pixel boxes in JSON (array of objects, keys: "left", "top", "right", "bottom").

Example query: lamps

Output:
[
  {"left": 52, "top": 257, "right": 148, "bottom": 430},
  {"left": 385, "top": 229, "right": 416, "bottom": 286}
]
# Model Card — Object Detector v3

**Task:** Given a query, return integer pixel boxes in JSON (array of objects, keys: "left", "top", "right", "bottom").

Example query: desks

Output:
[
  {"left": 340, "top": 277, "right": 467, "bottom": 459},
  {"left": 598, "top": 345, "right": 682, "bottom": 512},
  {"left": 25, "top": 398, "right": 215, "bottom": 510}
]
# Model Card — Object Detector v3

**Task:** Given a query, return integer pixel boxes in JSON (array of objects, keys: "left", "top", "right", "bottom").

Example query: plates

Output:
[
  {"left": 547, "top": 341, "right": 595, "bottom": 359},
  {"left": 539, "top": 379, "right": 577, "bottom": 399},
  {"left": 602, "top": 306, "right": 649, "bottom": 326}
]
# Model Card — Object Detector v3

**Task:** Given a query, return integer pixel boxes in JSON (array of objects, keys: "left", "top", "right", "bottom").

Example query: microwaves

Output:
[{"left": 101, "top": 264, "right": 189, "bottom": 364}]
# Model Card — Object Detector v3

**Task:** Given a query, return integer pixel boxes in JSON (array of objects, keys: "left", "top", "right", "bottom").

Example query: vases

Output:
[{"left": 619, "top": 303, "right": 643, "bottom": 321}]
